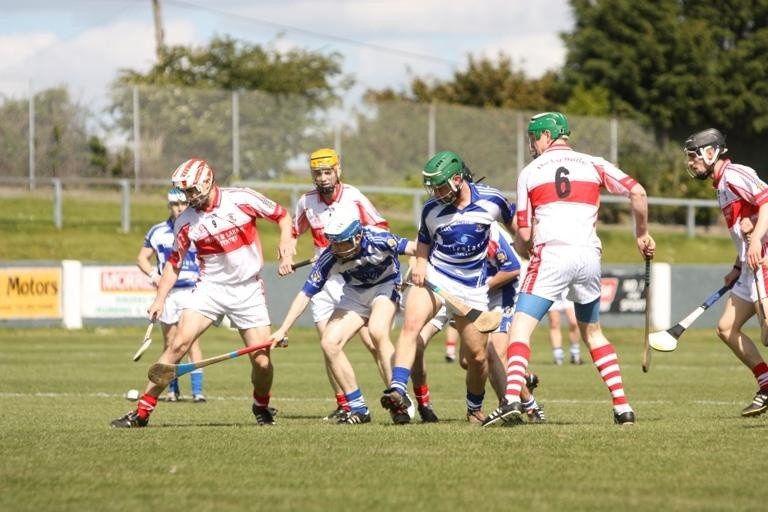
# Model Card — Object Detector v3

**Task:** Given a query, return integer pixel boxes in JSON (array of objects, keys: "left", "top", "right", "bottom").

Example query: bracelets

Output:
[{"left": 148, "top": 271, "right": 157, "bottom": 278}]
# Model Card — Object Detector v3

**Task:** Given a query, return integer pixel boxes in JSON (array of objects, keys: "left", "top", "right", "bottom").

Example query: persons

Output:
[
  {"left": 136, "top": 187, "right": 206, "bottom": 402},
  {"left": 409, "top": 235, "right": 539, "bottom": 425},
  {"left": 443, "top": 285, "right": 584, "bottom": 414},
  {"left": 481, "top": 113, "right": 656, "bottom": 428},
  {"left": 380, "top": 151, "right": 534, "bottom": 422},
  {"left": 277, "top": 150, "right": 389, "bottom": 419},
  {"left": 111, "top": 158, "right": 297, "bottom": 429},
  {"left": 265, "top": 214, "right": 418, "bottom": 424},
  {"left": 683, "top": 128, "right": 768, "bottom": 417}
]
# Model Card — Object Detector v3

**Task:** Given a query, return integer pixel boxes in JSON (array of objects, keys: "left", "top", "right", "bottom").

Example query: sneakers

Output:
[
  {"left": 335, "top": 408, "right": 370, "bottom": 425},
  {"left": 525, "top": 404, "right": 544, "bottom": 423},
  {"left": 192, "top": 395, "right": 205, "bottom": 403},
  {"left": 388, "top": 409, "right": 409, "bottom": 424},
  {"left": 324, "top": 407, "right": 348, "bottom": 422},
  {"left": 417, "top": 405, "right": 438, "bottom": 425},
  {"left": 481, "top": 397, "right": 522, "bottom": 427},
  {"left": 110, "top": 412, "right": 147, "bottom": 428},
  {"left": 526, "top": 374, "right": 538, "bottom": 393},
  {"left": 740, "top": 390, "right": 767, "bottom": 416},
  {"left": 165, "top": 393, "right": 178, "bottom": 402},
  {"left": 612, "top": 408, "right": 633, "bottom": 424},
  {"left": 252, "top": 403, "right": 277, "bottom": 426},
  {"left": 465, "top": 407, "right": 485, "bottom": 424},
  {"left": 380, "top": 387, "right": 410, "bottom": 415},
  {"left": 405, "top": 393, "right": 415, "bottom": 419}
]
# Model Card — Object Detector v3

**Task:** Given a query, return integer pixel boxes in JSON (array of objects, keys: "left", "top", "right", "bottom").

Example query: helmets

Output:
[
  {"left": 421, "top": 150, "right": 466, "bottom": 208},
  {"left": 683, "top": 128, "right": 728, "bottom": 180},
  {"left": 322, "top": 211, "right": 364, "bottom": 261},
  {"left": 309, "top": 148, "right": 340, "bottom": 183},
  {"left": 167, "top": 187, "right": 187, "bottom": 204},
  {"left": 526, "top": 112, "right": 570, "bottom": 140},
  {"left": 171, "top": 158, "right": 215, "bottom": 207}
]
